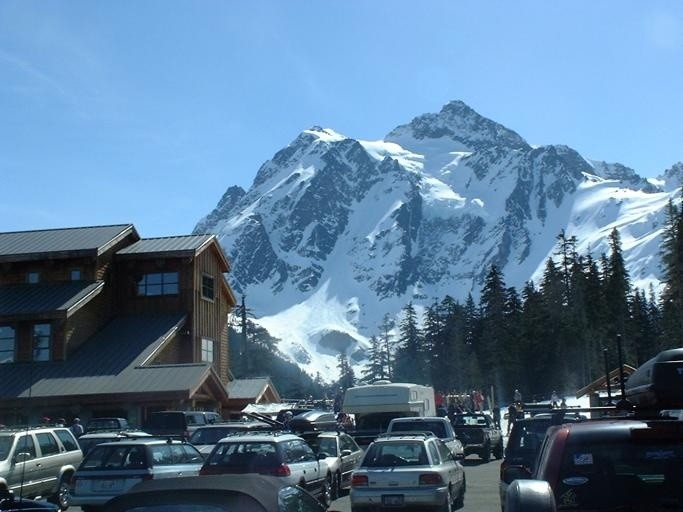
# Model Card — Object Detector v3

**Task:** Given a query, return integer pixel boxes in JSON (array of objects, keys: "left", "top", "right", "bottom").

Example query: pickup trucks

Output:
[
  {"left": 449, "top": 410, "right": 503, "bottom": 462},
  {"left": 388, "top": 416, "right": 464, "bottom": 467}
]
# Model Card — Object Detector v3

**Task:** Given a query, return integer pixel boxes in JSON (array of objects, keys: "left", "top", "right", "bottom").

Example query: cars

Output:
[
  {"left": 0, "top": 496, "right": 62, "bottom": 512},
  {"left": 74, "top": 427, "right": 150, "bottom": 454},
  {"left": 348, "top": 430, "right": 467, "bottom": 511},
  {"left": 289, "top": 411, "right": 365, "bottom": 498},
  {"left": 79, "top": 416, "right": 128, "bottom": 435},
  {"left": 100, "top": 471, "right": 328, "bottom": 512}
]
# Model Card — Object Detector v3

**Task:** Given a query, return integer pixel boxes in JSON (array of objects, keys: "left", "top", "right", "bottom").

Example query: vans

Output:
[
  {"left": 148, "top": 410, "right": 224, "bottom": 448},
  {"left": 188, "top": 420, "right": 274, "bottom": 458}
]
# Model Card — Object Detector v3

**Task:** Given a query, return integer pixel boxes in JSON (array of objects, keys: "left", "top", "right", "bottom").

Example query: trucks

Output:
[{"left": 341, "top": 379, "right": 436, "bottom": 456}]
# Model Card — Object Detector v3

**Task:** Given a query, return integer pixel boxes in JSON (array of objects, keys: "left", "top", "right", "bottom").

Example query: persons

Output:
[
  {"left": 493, "top": 402, "right": 501, "bottom": 430},
  {"left": 513, "top": 389, "right": 521, "bottom": 401},
  {"left": 506, "top": 403, "right": 518, "bottom": 437},
  {"left": 307, "top": 396, "right": 313, "bottom": 408},
  {"left": 283, "top": 411, "right": 314, "bottom": 433},
  {"left": 41, "top": 417, "right": 83, "bottom": 440},
  {"left": 435, "top": 389, "right": 483, "bottom": 415},
  {"left": 336, "top": 412, "right": 354, "bottom": 432},
  {"left": 550, "top": 391, "right": 560, "bottom": 407}
]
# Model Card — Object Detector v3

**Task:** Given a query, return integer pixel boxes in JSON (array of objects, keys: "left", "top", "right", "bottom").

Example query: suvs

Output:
[
  {"left": 504, "top": 334, "right": 683, "bottom": 512},
  {"left": 0, "top": 424, "right": 83, "bottom": 508},
  {"left": 68, "top": 434, "right": 204, "bottom": 511},
  {"left": 200, "top": 429, "right": 333, "bottom": 507},
  {"left": 500, "top": 413, "right": 593, "bottom": 512}
]
